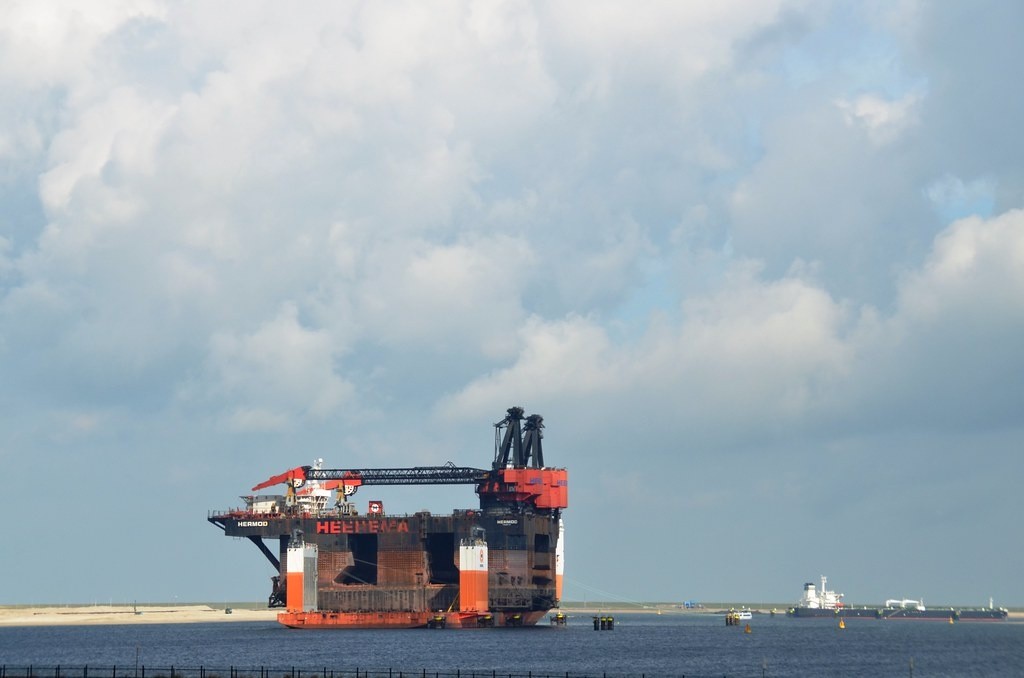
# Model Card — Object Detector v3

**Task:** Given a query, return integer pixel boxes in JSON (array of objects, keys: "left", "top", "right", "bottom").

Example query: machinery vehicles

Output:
[{"left": 250, "top": 405, "right": 570, "bottom": 513}]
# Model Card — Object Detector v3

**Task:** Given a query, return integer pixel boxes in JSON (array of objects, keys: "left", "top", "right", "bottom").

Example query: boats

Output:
[
  {"left": 732, "top": 611, "right": 753, "bottom": 620},
  {"left": 786, "top": 574, "right": 1009, "bottom": 623},
  {"left": 205, "top": 489, "right": 566, "bottom": 631}
]
